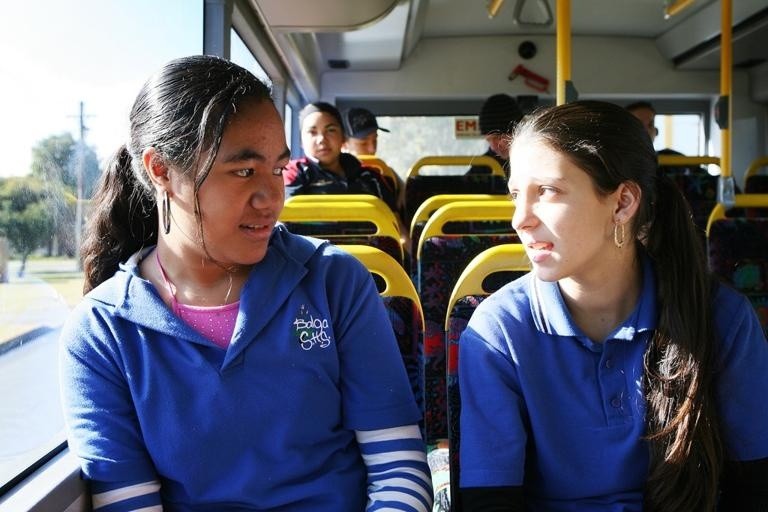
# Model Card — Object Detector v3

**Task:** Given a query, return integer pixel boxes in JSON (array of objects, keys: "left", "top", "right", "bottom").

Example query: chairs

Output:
[
  {"left": 280, "top": 153, "right": 535, "bottom": 510},
  {"left": 657, "top": 155, "right": 767, "bottom": 329}
]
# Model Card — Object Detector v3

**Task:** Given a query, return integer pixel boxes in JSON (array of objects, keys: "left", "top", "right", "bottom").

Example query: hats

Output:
[
  {"left": 341, "top": 107, "right": 391, "bottom": 140},
  {"left": 478, "top": 93, "right": 524, "bottom": 135}
]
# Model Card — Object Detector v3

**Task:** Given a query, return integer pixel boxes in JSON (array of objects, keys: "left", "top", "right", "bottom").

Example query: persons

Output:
[
  {"left": 464, "top": 92, "right": 524, "bottom": 175},
  {"left": 284, "top": 99, "right": 401, "bottom": 254},
  {"left": 340, "top": 106, "right": 407, "bottom": 207},
  {"left": 60, "top": 52, "right": 437, "bottom": 512},
  {"left": 455, "top": 97, "right": 768, "bottom": 511},
  {"left": 622, "top": 99, "right": 685, "bottom": 157}
]
